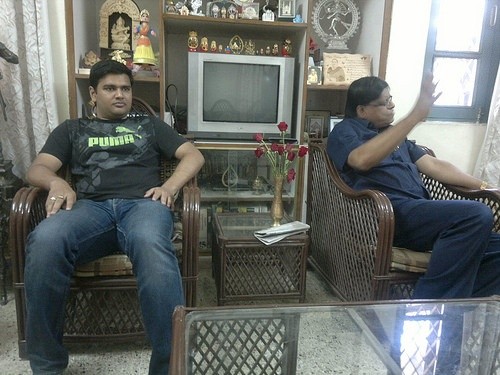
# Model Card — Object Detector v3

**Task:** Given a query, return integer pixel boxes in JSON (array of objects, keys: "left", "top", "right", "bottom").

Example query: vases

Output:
[{"left": 270, "top": 175, "right": 283, "bottom": 229}]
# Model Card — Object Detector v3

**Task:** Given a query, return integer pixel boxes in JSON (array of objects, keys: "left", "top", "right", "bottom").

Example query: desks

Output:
[{"left": 166, "top": 293, "right": 500, "bottom": 375}]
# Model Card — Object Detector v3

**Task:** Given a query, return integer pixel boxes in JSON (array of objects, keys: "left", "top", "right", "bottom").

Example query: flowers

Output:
[{"left": 253, "top": 120, "right": 310, "bottom": 184}]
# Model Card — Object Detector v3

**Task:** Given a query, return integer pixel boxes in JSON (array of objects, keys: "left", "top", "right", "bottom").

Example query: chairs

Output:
[
  {"left": 9, "top": 95, "right": 203, "bottom": 362},
  {"left": 305, "top": 140, "right": 500, "bottom": 302}
]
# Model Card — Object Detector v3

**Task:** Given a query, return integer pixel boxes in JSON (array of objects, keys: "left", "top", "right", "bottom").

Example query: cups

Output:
[
  {"left": 164, "top": 111, "right": 175, "bottom": 129},
  {"left": 308, "top": 57, "right": 314, "bottom": 66}
]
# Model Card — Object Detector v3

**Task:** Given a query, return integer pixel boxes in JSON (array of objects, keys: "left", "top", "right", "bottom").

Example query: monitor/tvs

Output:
[{"left": 186, "top": 52, "right": 295, "bottom": 142}]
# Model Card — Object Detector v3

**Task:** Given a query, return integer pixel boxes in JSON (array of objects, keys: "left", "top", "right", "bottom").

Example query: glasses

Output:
[{"left": 362, "top": 95, "right": 393, "bottom": 106}]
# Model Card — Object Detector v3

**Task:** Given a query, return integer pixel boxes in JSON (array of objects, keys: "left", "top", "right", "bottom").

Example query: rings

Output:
[
  {"left": 57, "top": 195, "right": 64, "bottom": 199},
  {"left": 49, "top": 197, "right": 57, "bottom": 201}
]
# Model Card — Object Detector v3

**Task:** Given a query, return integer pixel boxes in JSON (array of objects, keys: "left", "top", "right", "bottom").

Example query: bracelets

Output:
[{"left": 480, "top": 181, "right": 487, "bottom": 190}]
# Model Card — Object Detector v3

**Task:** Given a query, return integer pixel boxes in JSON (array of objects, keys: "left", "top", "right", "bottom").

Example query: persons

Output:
[
  {"left": 131, "top": 8, "right": 157, "bottom": 66},
  {"left": 326, "top": 71, "right": 500, "bottom": 299},
  {"left": 111, "top": 16, "right": 130, "bottom": 45},
  {"left": 25, "top": 60, "right": 205, "bottom": 375}
]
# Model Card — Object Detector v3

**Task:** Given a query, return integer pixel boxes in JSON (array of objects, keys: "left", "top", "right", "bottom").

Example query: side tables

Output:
[{"left": 209, "top": 212, "right": 310, "bottom": 307}]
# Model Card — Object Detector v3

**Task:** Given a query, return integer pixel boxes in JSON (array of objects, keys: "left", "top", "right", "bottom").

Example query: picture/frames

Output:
[
  {"left": 242, "top": 2, "right": 260, "bottom": 21},
  {"left": 305, "top": 110, "right": 331, "bottom": 143},
  {"left": 328, "top": 116, "right": 344, "bottom": 136},
  {"left": 277, "top": 0, "right": 297, "bottom": 19}
]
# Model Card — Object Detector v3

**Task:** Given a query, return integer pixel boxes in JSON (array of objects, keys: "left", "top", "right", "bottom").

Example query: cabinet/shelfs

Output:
[{"left": 66, "top": 0, "right": 396, "bottom": 224}]
